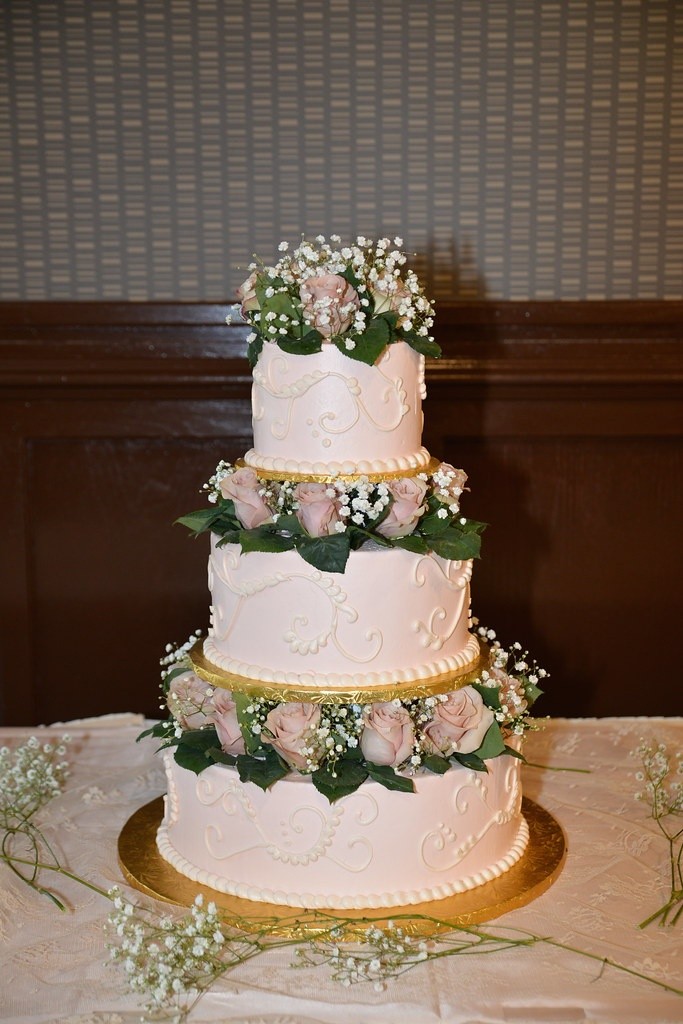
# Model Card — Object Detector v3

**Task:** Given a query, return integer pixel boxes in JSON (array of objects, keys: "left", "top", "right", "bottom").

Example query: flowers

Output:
[
  {"left": 226, "top": 227, "right": 443, "bottom": 366},
  {"left": 102, "top": 887, "right": 683, "bottom": 1024},
  {"left": 147, "top": 596, "right": 549, "bottom": 803},
  {"left": 624, "top": 737, "right": 683, "bottom": 930},
  {"left": 173, "top": 458, "right": 488, "bottom": 573},
  {"left": 0, "top": 731, "right": 112, "bottom": 912}
]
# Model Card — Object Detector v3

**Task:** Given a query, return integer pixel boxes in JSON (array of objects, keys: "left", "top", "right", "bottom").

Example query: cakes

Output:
[{"left": 134, "top": 234, "right": 549, "bottom": 910}]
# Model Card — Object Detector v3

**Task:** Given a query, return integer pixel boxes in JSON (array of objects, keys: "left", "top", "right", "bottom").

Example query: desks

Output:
[{"left": 0, "top": 714, "right": 683, "bottom": 1024}]
